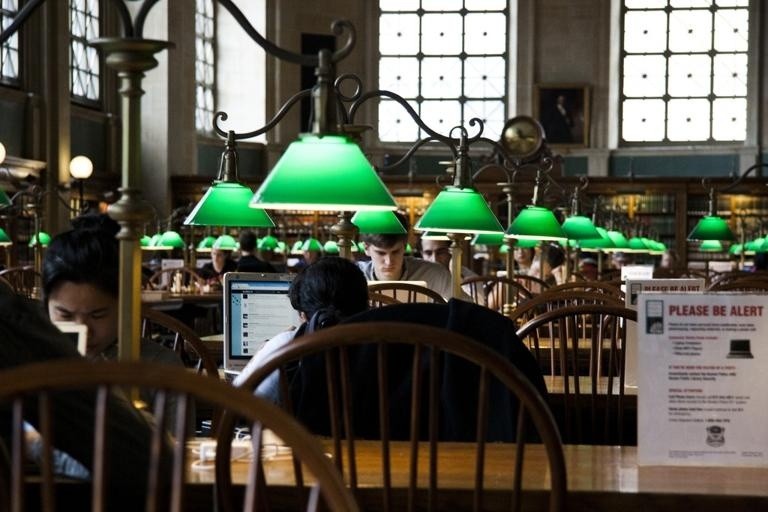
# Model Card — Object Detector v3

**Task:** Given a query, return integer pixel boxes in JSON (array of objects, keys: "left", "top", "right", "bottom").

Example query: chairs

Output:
[
  {"left": 599, "top": 270, "right": 622, "bottom": 283},
  {"left": 1, "top": 266, "right": 47, "bottom": 301},
  {"left": 707, "top": 272, "right": 768, "bottom": 293},
  {"left": 0, "top": 359, "right": 360, "bottom": 512},
  {"left": 512, "top": 303, "right": 639, "bottom": 418},
  {"left": 715, "top": 281, "right": 768, "bottom": 291},
  {"left": 366, "top": 292, "right": 398, "bottom": 310},
  {"left": 483, "top": 273, "right": 551, "bottom": 315},
  {"left": 367, "top": 282, "right": 448, "bottom": 305},
  {"left": 213, "top": 323, "right": 566, "bottom": 512},
  {"left": 545, "top": 273, "right": 588, "bottom": 293},
  {"left": 460, "top": 275, "right": 534, "bottom": 317},
  {"left": 511, "top": 291, "right": 626, "bottom": 378},
  {"left": 539, "top": 282, "right": 627, "bottom": 342},
  {"left": 0, "top": 276, "right": 17, "bottom": 296},
  {"left": 138, "top": 304, "right": 219, "bottom": 381},
  {"left": 142, "top": 273, "right": 156, "bottom": 292},
  {"left": 147, "top": 269, "right": 208, "bottom": 290}
]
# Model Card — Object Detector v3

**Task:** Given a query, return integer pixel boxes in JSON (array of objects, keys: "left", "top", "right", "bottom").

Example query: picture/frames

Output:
[{"left": 530, "top": 81, "right": 593, "bottom": 148}]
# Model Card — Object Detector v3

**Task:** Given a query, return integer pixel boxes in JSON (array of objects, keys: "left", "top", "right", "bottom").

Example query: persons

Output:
[
  {"left": 294, "top": 249, "right": 320, "bottom": 270},
  {"left": 0, "top": 277, "right": 188, "bottom": 493},
  {"left": 578, "top": 249, "right": 599, "bottom": 274},
  {"left": 660, "top": 252, "right": 675, "bottom": 268},
  {"left": 416, "top": 228, "right": 484, "bottom": 306},
  {"left": 197, "top": 249, "right": 235, "bottom": 284},
  {"left": 354, "top": 229, "right": 454, "bottom": 308},
  {"left": 229, "top": 257, "right": 371, "bottom": 406},
  {"left": 511, "top": 244, "right": 557, "bottom": 290},
  {"left": 486, "top": 239, "right": 565, "bottom": 310},
  {"left": 234, "top": 231, "right": 274, "bottom": 274},
  {"left": 551, "top": 95, "right": 576, "bottom": 144},
  {"left": 612, "top": 248, "right": 635, "bottom": 270},
  {"left": 40, "top": 213, "right": 196, "bottom": 442}
]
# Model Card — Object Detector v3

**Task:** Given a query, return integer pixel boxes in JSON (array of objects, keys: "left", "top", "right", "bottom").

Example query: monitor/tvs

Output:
[
  {"left": 706, "top": 259, "right": 760, "bottom": 280},
  {"left": 223, "top": 273, "right": 304, "bottom": 374}
]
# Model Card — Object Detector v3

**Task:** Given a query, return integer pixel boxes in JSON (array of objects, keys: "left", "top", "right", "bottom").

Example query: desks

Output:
[
  {"left": 16, "top": 430, "right": 768, "bottom": 511},
  {"left": 22, "top": 286, "right": 185, "bottom": 343},
  {"left": 185, "top": 364, "right": 641, "bottom": 403},
  {"left": 169, "top": 290, "right": 225, "bottom": 306},
  {"left": 183, "top": 330, "right": 623, "bottom": 355}
]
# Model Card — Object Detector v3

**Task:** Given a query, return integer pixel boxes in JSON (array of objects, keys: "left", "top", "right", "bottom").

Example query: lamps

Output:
[
  {"left": 150, "top": 232, "right": 162, "bottom": 247},
  {"left": 626, "top": 222, "right": 648, "bottom": 254},
  {"left": 158, "top": 216, "right": 186, "bottom": 248},
  {"left": 405, "top": 243, "right": 412, "bottom": 253},
  {"left": 685, "top": 187, "right": 738, "bottom": 243},
  {"left": 560, "top": 185, "right": 603, "bottom": 242},
  {"left": 579, "top": 197, "right": 618, "bottom": 250},
  {"left": 350, "top": 240, "right": 358, "bottom": 252},
  {"left": 728, "top": 227, "right": 768, "bottom": 256},
  {"left": 506, "top": 169, "right": 568, "bottom": 241},
  {"left": 357, "top": 241, "right": 366, "bottom": 252},
  {"left": 290, "top": 241, "right": 303, "bottom": 254},
  {"left": 324, "top": 241, "right": 338, "bottom": 254},
  {"left": 0, "top": 186, "right": 13, "bottom": 208},
  {"left": 641, "top": 223, "right": 652, "bottom": 254},
  {"left": 196, "top": 235, "right": 216, "bottom": 252},
  {"left": 607, "top": 210, "right": 630, "bottom": 255},
  {"left": 0, "top": 227, "right": 14, "bottom": 246},
  {"left": 28, "top": 233, "right": 54, "bottom": 247},
  {"left": 301, "top": 237, "right": 323, "bottom": 252},
  {"left": 181, "top": 129, "right": 277, "bottom": 229},
  {"left": 511, "top": 238, "right": 541, "bottom": 249},
  {"left": 650, "top": 238, "right": 658, "bottom": 254},
  {"left": 420, "top": 231, "right": 450, "bottom": 242},
  {"left": 697, "top": 239, "right": 723, "bottom": 250},
  {"left": 1, "top": 144, "right": 5, "bottom": 164},
  {"left": 468, "top": 233, "right": 508, "bottom": 247},
  {"left": 213, "top": 234, "right": 237, "bottom": 252},
  {"left": 140, "top": 234, "right": 151, "bottom": 247},
  {"left": 410, "top": 127, "right": 507, "bottom": 237},
  {"left": 259, "top": 236, "right": 279, "bottom": 251},
  {"left": 658, "top": 242, "right": 666, "bottom": 255},
  {"left": 69, "top": 155, "right": 94, "bottom": 212},
  {"left": 247, "top": 50, "right": 401, "bottom": 213}
]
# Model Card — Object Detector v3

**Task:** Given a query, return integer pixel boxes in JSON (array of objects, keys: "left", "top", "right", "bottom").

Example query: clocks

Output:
[{"left": 502, "top": 115, "right": 546, "bottom": 163}]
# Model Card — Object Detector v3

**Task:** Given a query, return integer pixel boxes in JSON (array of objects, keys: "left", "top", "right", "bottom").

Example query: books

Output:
[
  {"left": 686, "top": 234, "right": 767, "bottom": 248},
  {"left": 173, "top": 215, "right": 273, "bottom": 236},
  {"left": 393, "top": 193, "right": 429, "bottom": 250},
  {"left": 273, "top": 219, "right": 338, "bottom": 236},
  {"left": 0, "top": 193, "right": 81, "bottom": 288},
  {"left": 574, "top": 191, "right": 675, "bottom": 214},
  {"left": 271, "top": 208, "right": 340, "bottom": 216},
  {"left": 594, "top": 215, "right": 675, "bottom": 236},
  {"left": 686, "top": 219, "right": 767, "bottom": 235},
  {"left": 688, "top": 245, "right": 729, "bottom": 260},
  {"left": 649, "top": 236, "right": 675, "bottom": 252},
  {"left": 686, "top": 192, "right": 767, "bottom": 218}
]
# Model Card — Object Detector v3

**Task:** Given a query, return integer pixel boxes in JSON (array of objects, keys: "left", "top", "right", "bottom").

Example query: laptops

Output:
[
  {"left": 725, "top": 338, "right": 754, "bottom": 360},
  {"left": 56, "top": 324, "right": 88, "bottom": 357}
]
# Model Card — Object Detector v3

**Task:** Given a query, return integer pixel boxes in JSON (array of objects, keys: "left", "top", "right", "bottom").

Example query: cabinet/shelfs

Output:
[
  {"left": 569, "top": 176, "right": 683, "bottom": 265},
  {"left": 470, "top": 178, "right": 569, "bottom": 238},
  {"left": 170, "top": 175, "right": 359, "bottom": 245},
  {"left": 683, "top": 176, "right": 767, "bottom": 275},
  {"left": 0, "top": 180, "right": 117, "bottom": 296},
  {"left": 376, "top": 174, "right": 452, "bottom": 247}
]
